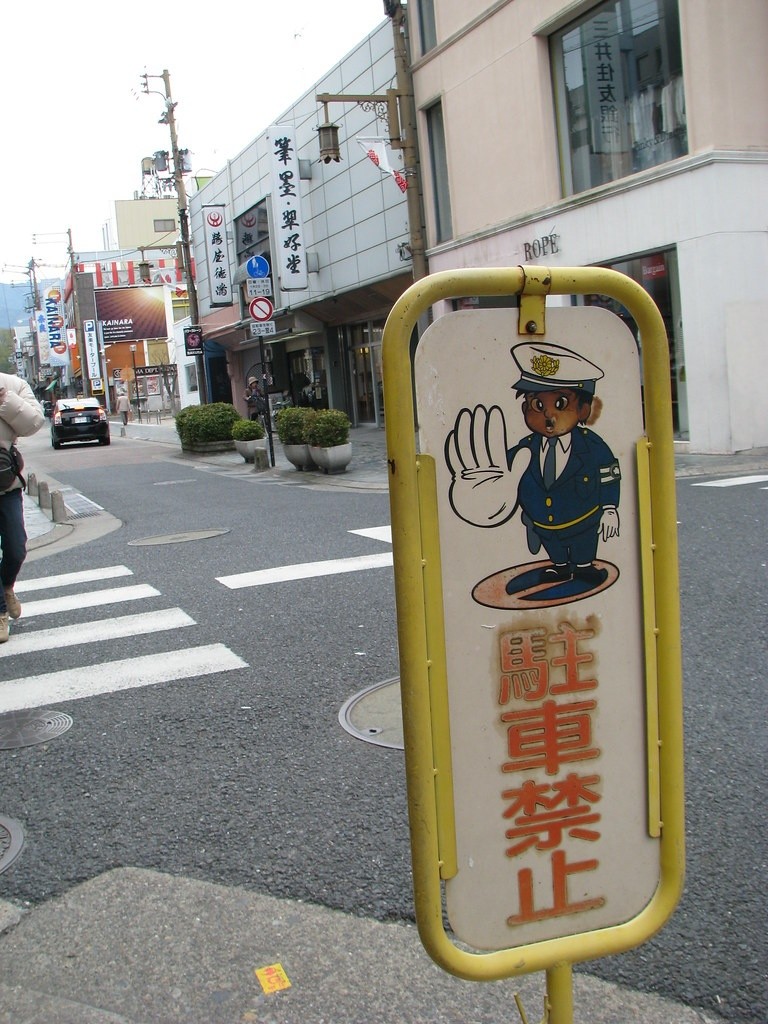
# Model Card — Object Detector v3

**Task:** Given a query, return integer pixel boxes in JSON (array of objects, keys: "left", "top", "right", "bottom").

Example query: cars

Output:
[{"left": 50, "top": 398, "right": 111, "bottom": 450}]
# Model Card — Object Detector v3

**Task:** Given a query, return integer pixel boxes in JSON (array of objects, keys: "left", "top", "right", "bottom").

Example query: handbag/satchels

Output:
[{"left": 0, "top": 448, "right": 27, "bottom": 492}]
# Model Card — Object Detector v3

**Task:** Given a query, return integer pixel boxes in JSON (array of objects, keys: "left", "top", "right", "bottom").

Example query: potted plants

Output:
[
  {"left": 231, "top": 421, "right": 267, "bottom": 464},
  {"left": 303, "top": 409, "right": 353, "bottom": 475},
  {"left": 175, "top": 402, "right": 240, "bottom": 453},
  {"left": 276, "top": 406, "right": 318, "bottom": 471}
]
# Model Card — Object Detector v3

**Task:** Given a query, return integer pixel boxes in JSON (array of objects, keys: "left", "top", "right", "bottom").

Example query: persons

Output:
[
  {"left": 0, "top": 371, "right": 46, "bottom": 643},
  {"left": 241, "top": 377, "right": 265, "bottom": 422},
  {"left": 116, "top": 392, "right": 131, "bottom": 425}
]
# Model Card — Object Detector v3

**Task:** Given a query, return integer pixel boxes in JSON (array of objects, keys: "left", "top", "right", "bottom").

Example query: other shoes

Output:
[
  {"left": 1, "top": 581, "right": 21, "bottom": 619},
  {"left": 0, "top": 612, "right": 9, "bottom": 643}
]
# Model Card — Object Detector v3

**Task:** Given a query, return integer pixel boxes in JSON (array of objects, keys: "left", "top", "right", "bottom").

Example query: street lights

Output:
[
  {"left": 316, "top": 90, "right": 437, "bottom": 341},
  {"left": 129, "top": 344, "right": 142, "bottom": 423},
  {"left": 137, "top": 240, "right": 212, "bottom": 406}
]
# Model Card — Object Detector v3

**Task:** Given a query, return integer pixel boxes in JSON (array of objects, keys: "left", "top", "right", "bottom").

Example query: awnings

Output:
[{"left": 45, "top": 380, "right": 57, "bottom": 390}]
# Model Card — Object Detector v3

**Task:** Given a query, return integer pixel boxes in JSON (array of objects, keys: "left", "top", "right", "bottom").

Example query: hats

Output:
[{"left": 248, "top": 377, "right": 259, "bottom": 385}]
